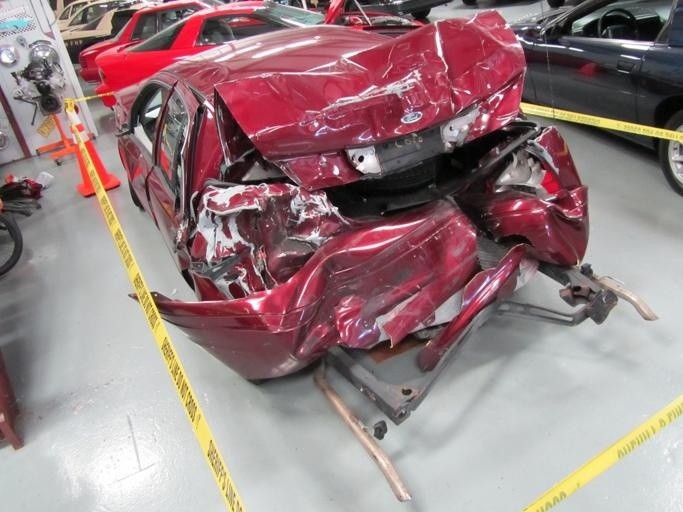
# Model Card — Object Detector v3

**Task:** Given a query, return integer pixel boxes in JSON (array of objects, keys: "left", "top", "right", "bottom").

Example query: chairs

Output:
[{"left": 207, "top": 17, "right": 235, "bottom": 43}]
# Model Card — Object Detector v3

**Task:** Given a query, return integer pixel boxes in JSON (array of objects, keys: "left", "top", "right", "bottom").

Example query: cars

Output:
[
  {"left": 426, "top": 0, "right": 561, "bottom": 26},
  {"left": 272, "top": 0, "right": 451, "bottom": 20},
  {"left": 55, "top": 0, "right": 211, "bottom": 83},
  {"left": 506, "top": 0, "right": 683, "bottom": 197},
  {"left": 111, "top": 10, "right": 591, "bottom": 380},
  {"left": 92, "top": 0, "right": 426, "bottom": 112}
]
